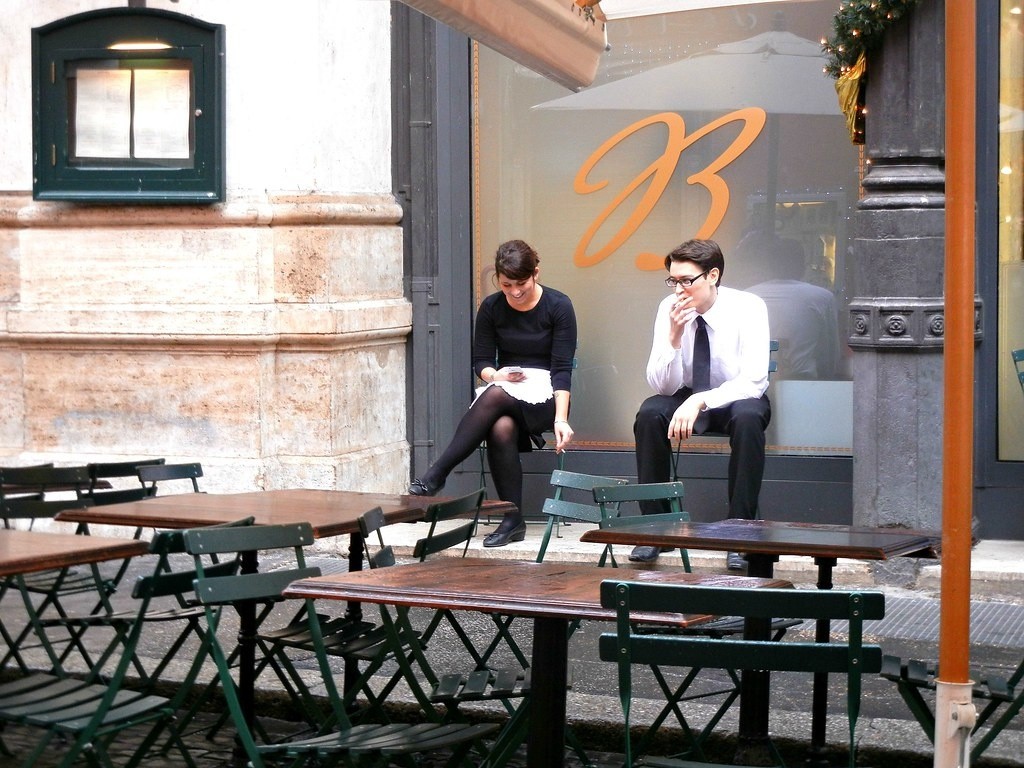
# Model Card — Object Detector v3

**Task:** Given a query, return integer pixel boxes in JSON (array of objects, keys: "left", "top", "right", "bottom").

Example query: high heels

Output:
[
  {"left": 409, "top": 468, "right": 445, "bottom": 496},
  {"left": 483, "top": 520, "right": 526, "bottom": 546}
]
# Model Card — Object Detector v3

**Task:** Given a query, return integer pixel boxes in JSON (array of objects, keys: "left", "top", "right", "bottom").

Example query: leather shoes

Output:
[
  {"left": 628, "top": 544, "right": 675, "bottom": 562},
  {"left": 727, "top": 550, "right": 747, "bottom": 570}
]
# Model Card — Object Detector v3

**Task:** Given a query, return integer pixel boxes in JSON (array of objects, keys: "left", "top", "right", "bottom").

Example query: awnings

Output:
[{"left": 400, "top": 0, "right": 612, "bottom": 93}]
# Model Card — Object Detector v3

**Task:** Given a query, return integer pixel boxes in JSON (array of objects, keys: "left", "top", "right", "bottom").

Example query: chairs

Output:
[
  {"left": 0, "top": 452, "right": 1024, "bottom": 768},
  {"left": 666, "top": 339, "right": 779, "bottom": 518},
  {"left": 476, "top": 355, "right": 579, "bottom": 538}
]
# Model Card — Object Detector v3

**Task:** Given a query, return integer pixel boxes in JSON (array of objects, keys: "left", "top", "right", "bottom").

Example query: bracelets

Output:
[
  {"left": 489, "top": 372, "right": 495, "bottom": 382},
  {"left": 554, "top": 420, "right": 568, "bottom": 424}
]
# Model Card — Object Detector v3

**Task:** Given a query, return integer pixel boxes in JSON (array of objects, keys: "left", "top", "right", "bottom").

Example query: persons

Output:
[
  {"left": 741, "top": 239, "right": 842, "bottom": 381},
  {"left": 409, "top": 240, "right": 578, "bottom": 547},
  {"left": 630, "top": 239, "right": 771, "bottom": 569}
]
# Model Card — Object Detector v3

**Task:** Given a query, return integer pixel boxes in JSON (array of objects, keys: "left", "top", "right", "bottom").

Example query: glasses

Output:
[{"left": 665, "top": 268, "right": 711, "bottom": 287}]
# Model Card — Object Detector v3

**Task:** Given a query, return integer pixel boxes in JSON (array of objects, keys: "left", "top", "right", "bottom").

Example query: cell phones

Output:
[{"left": 508, "top": 368, "right": 523, "bottom": 375}]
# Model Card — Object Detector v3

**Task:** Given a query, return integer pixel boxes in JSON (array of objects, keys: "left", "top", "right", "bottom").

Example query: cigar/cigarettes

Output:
[
  {"left": 560, "top": 449, "right": 565, "bottom": 453},
  {"left": 675, "top": 300, "right": 684, "bottom": 307}
]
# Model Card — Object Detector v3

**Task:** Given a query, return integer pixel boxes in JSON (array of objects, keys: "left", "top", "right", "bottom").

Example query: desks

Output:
[
  {"left": 577, "top": 512, "right": 979, "bottom": 768},
  {"left": 50, "top": 493, "right": 423, "bottom": 718},
  {"left": 226, "top": 481, "right": 519, "bottom": 712},
  {"left": 282, "top": 549, "right": 793, "bottom": 767},
  {"left": 0, "top": 527, "right": 150, "bottom": 576}
]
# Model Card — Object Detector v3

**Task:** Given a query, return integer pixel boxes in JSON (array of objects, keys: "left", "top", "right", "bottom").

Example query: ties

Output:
[{"left": 692, "top": 315, "right": 710, "bottom": 434}]
